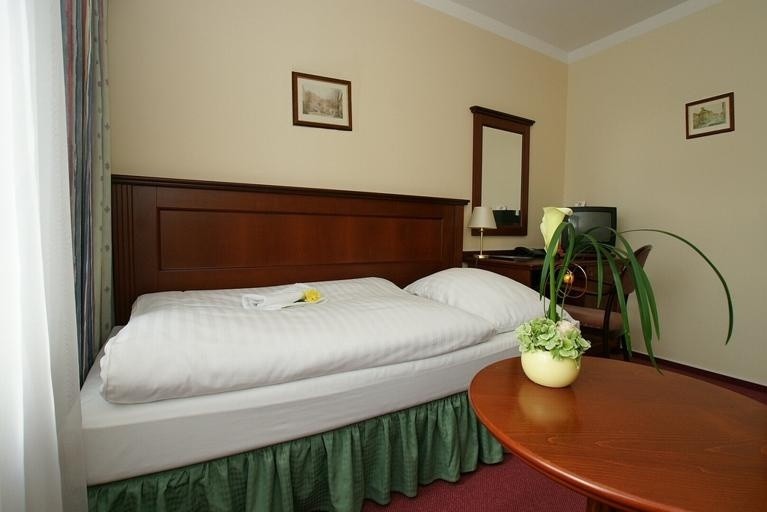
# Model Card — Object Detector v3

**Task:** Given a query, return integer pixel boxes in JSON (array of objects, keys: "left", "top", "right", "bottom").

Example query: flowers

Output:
[
  {"left": 295, "top": 287, "right": 322, "bottom": 303},
  {"left": 514, "top": 205, "right": 734, "bottom": 370}
]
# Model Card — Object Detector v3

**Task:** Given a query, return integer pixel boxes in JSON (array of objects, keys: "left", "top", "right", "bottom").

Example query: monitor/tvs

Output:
[{"left": 562, "top": 207, "right": 617, "bottom": 253}]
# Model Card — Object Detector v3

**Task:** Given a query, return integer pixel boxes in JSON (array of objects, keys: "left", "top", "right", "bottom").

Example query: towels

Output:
[{"left": 241, "top": 282, "right": 327, "bottom": 310}]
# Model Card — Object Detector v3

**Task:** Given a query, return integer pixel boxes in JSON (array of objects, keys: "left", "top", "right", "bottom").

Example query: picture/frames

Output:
[
  {"left": 291, "top": 71, "right": 353, "bottom": 131},
  {"left": 685, "top": 92, "right": 735, "bottom": 140}
]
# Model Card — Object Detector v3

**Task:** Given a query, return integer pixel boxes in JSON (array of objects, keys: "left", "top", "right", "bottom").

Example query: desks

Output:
[
  {"left": 472, "top": 254, "right": 630, "bottom": 352},
  {"left": 465, "top": 354, "right": 766, "bottom": 512}
]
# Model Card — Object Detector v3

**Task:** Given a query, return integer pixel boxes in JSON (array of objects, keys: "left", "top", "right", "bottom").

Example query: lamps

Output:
[{"left": 467, "top": 204, "right": 498, "bottom": 259}]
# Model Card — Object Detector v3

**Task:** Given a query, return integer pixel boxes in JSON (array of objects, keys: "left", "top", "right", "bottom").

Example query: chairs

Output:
[{"left": 560, "top": 244, "right": 652, "bottom": 361}]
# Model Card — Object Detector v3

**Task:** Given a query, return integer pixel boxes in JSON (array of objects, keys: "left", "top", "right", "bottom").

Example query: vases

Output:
[{"left": 520, "top": 349, "right": 580, "bottom": 388}]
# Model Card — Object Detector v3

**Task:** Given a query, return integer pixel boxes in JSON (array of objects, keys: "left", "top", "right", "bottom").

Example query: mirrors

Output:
[{"left": 468, "top": 105, "right": 536, "bottom": 237}]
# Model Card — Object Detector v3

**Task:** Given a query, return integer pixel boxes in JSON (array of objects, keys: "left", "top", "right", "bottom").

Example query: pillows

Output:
[{"left": 403, "top": 261, "right": 576, "bottom": 336}]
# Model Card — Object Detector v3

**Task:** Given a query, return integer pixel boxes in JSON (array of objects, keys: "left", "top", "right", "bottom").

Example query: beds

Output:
[{"left": 78, "top": 173, "right": 579, "bottom": 512}]
